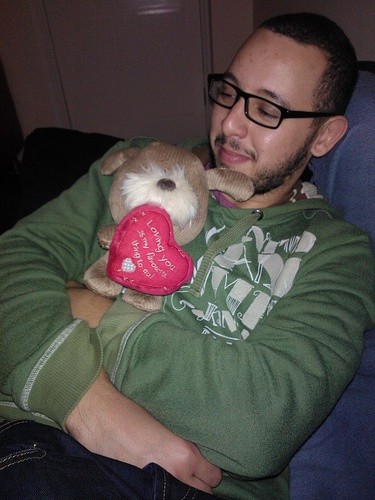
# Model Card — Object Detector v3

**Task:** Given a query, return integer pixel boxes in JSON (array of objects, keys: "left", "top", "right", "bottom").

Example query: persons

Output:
[{"left": 1, "top": 13, "right": 375, "bottom": 499}]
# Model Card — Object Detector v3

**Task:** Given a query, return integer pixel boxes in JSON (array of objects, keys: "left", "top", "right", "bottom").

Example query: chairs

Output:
[{"left": 290, "top": 70, "right": 375, "bottom": 500}]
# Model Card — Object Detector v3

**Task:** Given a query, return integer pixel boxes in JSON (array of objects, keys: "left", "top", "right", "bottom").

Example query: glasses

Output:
[{"left": 205, "top": 71, "right": 335, "bottom": 131}]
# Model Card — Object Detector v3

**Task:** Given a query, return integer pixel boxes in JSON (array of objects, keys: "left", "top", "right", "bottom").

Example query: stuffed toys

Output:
[{"left": 84, "top": 142, "right": 256, "bottom": 311}]
